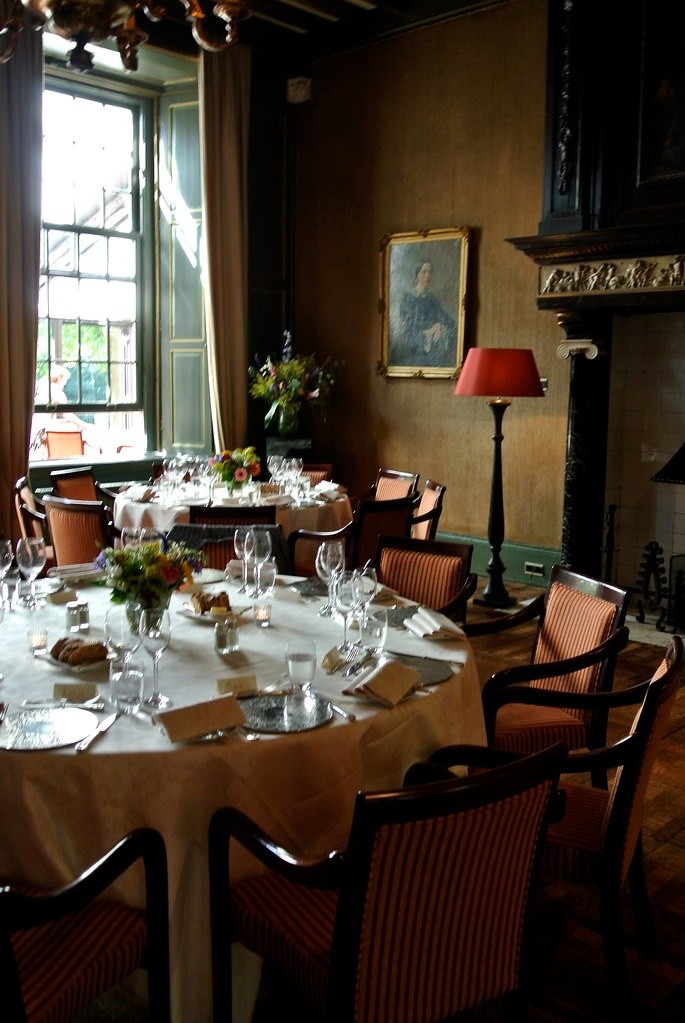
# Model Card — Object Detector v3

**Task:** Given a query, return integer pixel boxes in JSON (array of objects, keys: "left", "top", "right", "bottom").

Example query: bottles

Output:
[
  {"left": 66, "top": 600, "right": 89, "bottom": 633},
  {"left": 216, "top": 619, "right": 240, "bottom": 653}
]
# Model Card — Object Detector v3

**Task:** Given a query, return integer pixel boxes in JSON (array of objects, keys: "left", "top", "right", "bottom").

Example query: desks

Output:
[
  {"left": 113, "top": 478, "right": 352, "bottom": 578},
  {"left": 0, "top": 572, "right": 488, "bottom": 1023}
]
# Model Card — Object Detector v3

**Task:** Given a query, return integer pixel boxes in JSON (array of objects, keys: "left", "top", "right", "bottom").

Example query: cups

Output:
[
  {"left": 284, "top": 635, "right": 316, "bottom": 687},
  {"left": 359, "top": 607, "right": 387, "bottom": 656},
  {"left": 108, "top": 657, "right": 145, "bottom": 713},
  {"left": 121, "top": 525, "right": 162, "bottom": 555},
  {"left": 253, "top": 562, "right": 276, "bottom": 628}
]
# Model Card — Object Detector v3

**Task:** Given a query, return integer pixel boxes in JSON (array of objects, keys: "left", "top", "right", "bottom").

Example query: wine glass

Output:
[
  {"left": 106, "top": 602, "right": 174, "bottom": 713},
  {"left": 234, "top": 529, "right": 271, "bottom": 598},
  {"left": 316, "top": 541, "right": 377, "bottom": 657},
  {"left": 0, "top": 538, "right": 46, "bottom": 622},
  {"left": 127, "top": 452, "right": 313, "bottom": 506}
]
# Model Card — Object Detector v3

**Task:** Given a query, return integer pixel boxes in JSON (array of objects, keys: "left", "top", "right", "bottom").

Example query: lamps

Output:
[
  {"left": 455, "top": 347, "right": 545, "bottom": 608},
  {"left": 0, "top": 1, "right": 249, "bottom": 76}
]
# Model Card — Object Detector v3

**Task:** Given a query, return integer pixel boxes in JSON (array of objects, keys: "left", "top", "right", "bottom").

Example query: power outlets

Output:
[{"left": 525, "top": 562, "right": 545, "bottom": 578}]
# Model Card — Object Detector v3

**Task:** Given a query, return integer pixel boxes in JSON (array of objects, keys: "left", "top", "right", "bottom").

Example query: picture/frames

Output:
[{"left": 374, "top": 224, "right": 471, "bottom": 378}]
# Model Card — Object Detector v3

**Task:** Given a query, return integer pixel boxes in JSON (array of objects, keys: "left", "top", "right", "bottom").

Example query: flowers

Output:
[
  {"left": 95, "top": 537, "right": 208, "bottom": 632},
  {"left": 245, "top": 328, "right": 345, "bottom": 430},
  {"left": 209, "top": 447, "right": 262, "bottom": 494}
]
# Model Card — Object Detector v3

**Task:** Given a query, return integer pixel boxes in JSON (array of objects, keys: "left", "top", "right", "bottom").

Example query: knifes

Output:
[
  {"left": 347, "top": 648, "right": 375, "bottom": 677},
  {"left": 75, "top": 713, "right": 117, "bottom": 751}
]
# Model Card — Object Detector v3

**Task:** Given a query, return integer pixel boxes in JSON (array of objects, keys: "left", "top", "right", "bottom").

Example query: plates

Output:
[
  {"left": 291, "top": 578, "right": 330, "bottom": 597},
  {"left": 34, "top": 578, "right": 66, "bottom": 597},
  {"left": 0, "top": 707, "right": 99, "bottom": 749},
  {"left": 185, "top": 567, "right": 225, "bottom": 583},
  {"left": 363, "top": 653, "right": 454, "bottom": 685},
  {"left": 387, "top": 604, "right": 430, "bottom": 629},
  {"left": 174, "top": 603, "right": 252, "bottom": 624},
  {"left": 238, "top": 691, "right": 332, "bottom": 731}
]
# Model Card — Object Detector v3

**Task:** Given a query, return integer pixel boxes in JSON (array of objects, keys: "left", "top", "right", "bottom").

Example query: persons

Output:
[{"left": 37, "top": 364, "right": 94, "bottom": 428}]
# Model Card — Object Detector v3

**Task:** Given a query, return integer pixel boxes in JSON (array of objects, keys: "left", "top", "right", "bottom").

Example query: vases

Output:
[
  {"left": 278, "top": 410, "right": 300, "bottom": 439},
  {"left": 225, "top": 480, "right": 249, "bottom": 498},
  {"left": 124, "top": 598, "right": 165, "bottom": 637}
]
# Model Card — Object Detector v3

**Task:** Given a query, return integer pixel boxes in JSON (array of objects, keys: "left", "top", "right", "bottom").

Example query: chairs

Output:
[
  {"left": 168, "top": 522, "right": 285, "bottom": 575},
  {"left": 290, "top": 469, "right": 673, "bottom": 1023},
  {"left": 188, "top": 505, "right": 276, "bottom": 526},
  {"left": 0, "top": 825, "right": 170, "bottom": 1023},
  {"left": 16, "top": 432, "right": 154, "bottom": 579},
  {"left": 209, "top": 741, "right": 571, "bottom": 1023}
]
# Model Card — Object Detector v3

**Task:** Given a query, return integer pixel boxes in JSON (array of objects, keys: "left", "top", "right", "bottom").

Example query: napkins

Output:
[{"left": 0, "top": 485, "right": 464, "bottom": 744}]
